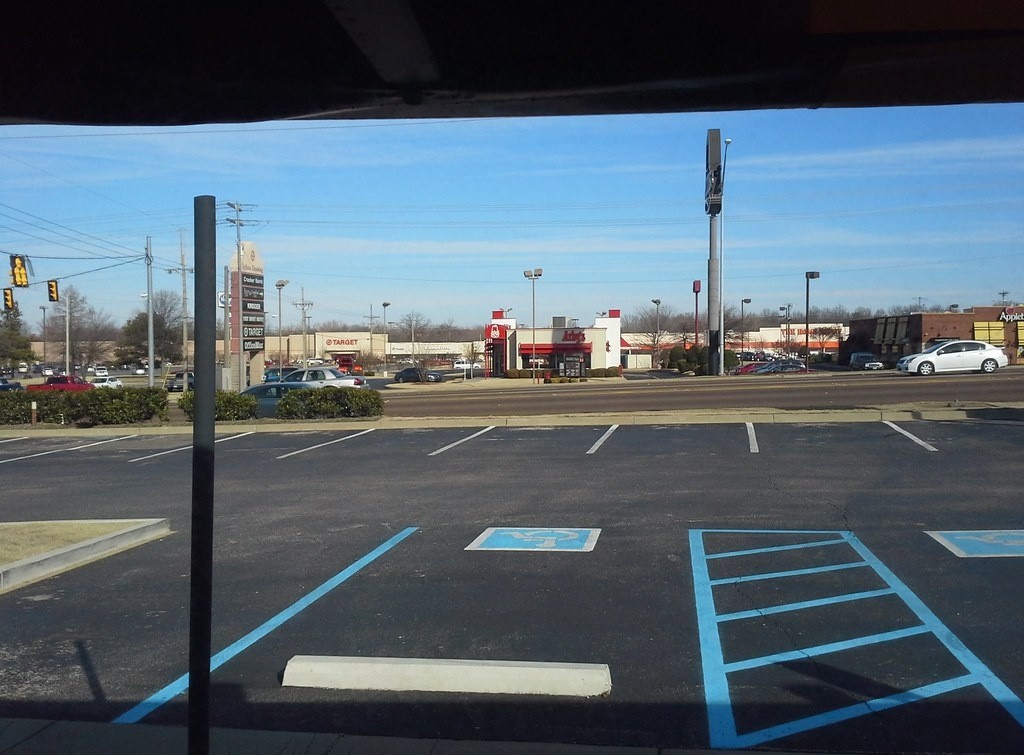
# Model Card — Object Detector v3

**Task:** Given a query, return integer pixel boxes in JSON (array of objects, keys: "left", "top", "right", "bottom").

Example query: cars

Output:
[
  {"left": 233, "top": 382, "right": 315, "bottom": 418},
  {"left": 733, "top": 351, "right": 806, "bottom": 376},
  {"left": 247, "top": 356, "right": 326, "bottom": 386},
  {"left": 18, "top": 362, "right": 28, "bottom": 373},
  {"left": 30, "top": 363, "right": 66, "bottom": 376},
  {"left": 88, "top": 376, "right": 124, "bottom": 391},
  {"left": 278, "top": 366, "right": 370, "bottom": 389},
  {"left": 895, "top": 338, "right": 1009, "bottom": 376},
  {"left": 394, "top": 367, "right": 442, "bottom": 383},
  {"left": 453, "top": 359, "right": 482, "bottom": 370},
  {"left": 0, "top": 364, "right": 15, "bottom": 378},
  {"left": 74, "top": 361, "right": 149, "bottom": 377},
  {"left": 0, "top": 377, "right": 24, "bottom": 392},
  {"left": 847, "top": 351, "right": 885, "bottom": 371}
]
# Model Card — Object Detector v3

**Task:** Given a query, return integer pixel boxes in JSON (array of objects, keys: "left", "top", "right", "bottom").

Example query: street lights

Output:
[
  {"left": 742, "top": 298, "right": 751, "bottom": 365},
  {"left": 383, "top": 302, "right": 391, "bottom": 377},
  {"left": 651, "top": 299, "right": 661, "bottom": 363},
  {"left": 276, "top": 279, "right": 290, "bottom": 381},
  {"left": 805, "top": 272, "right": 820, "bottom": 374},
  {"left": 718, "top": 138, "right": 732, "bottom": 376},
  {"left": 524, "top": 268, "right": 543, "bottom": 386},
  {"left": 39, "top": 305, "right": 49, "bottom": 384},
  {"left": 779, "top": 307, "right": 788, "bottom": 359}
]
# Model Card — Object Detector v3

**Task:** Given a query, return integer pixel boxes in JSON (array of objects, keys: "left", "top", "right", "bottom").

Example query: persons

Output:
[{"left": 754, "top": 351, "right": 761, "bottom": 362}]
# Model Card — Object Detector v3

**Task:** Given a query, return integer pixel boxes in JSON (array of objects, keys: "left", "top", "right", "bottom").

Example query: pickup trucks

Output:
[
  {"left": 27, "top": 375, "right": 95, "bottom": 391},
  {"left": 164, "top": 371, "right": 194, "bottom": 392}
]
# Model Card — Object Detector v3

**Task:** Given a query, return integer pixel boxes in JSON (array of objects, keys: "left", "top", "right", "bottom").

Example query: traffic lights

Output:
[
  {"left": 4, "top": 289, "right": 13, "bottom": 309},
  {"left": 48, "top": 281, "right": 59, "bottom": 301}
]
